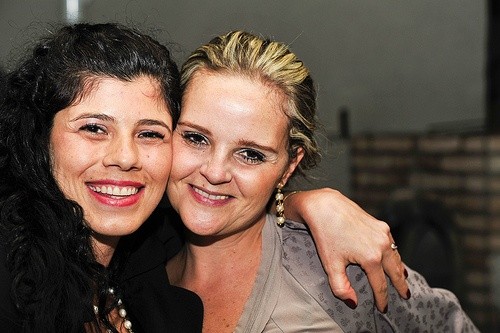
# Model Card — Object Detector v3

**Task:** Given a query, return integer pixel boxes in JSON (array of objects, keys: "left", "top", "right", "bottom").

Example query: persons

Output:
[
  {"left": 163, "top": 29, "right": 481, "bottom": 333},
  {"left": 0, "top": 21, "right": 412, "bottom": 332}
]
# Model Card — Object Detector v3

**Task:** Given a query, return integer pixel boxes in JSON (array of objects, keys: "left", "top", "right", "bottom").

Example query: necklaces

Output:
[{"left": 92, "top": 286, "right": 132, "bottom": 333}]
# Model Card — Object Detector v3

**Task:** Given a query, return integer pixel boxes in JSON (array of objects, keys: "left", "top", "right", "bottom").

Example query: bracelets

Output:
[{"left": 281, "top": 190, "right": 302, "bottom": 219}]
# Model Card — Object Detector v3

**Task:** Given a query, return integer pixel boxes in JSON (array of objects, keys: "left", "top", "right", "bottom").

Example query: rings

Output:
[{"left": 391, "top": 242, "right": 398, "bottom": 250}]
